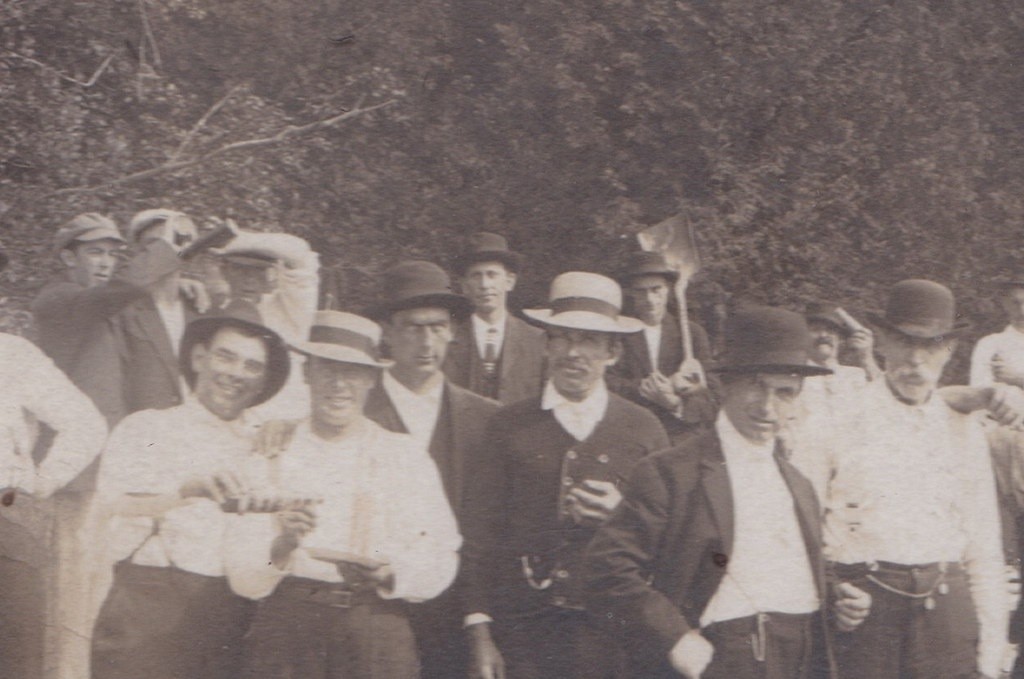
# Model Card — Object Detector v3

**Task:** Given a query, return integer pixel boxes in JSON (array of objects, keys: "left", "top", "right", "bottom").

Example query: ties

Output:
[{"left": 481, "top": 327, "right": 497, "bottom": 392}]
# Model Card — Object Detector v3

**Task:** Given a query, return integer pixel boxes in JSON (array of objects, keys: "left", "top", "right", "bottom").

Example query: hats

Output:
[
  {"left": 450, "top": 233, "right": 525, "bottom": 275},
  {"left": 997, "top": 274, "right": 1024, "bottom": 285},
  {"left": 176, "top": 298, "right": 290, "bottom": 409},
  {"left": 612, "top": 251, "right": 681, "bottom": 283},
  {"left": 522, "top": 270, "right": 645, "bottom": 333},
  {"left": 225, "top": 250, "right": 279, "bottom": 267},
  {"left": 131, "top": 209, "right": 188, "bottom": 242},
  {"left": 51, "top": 208, "right": 129, "bottom": 256},
  {"left": 866, "top": 280, "right": 966, "bottom": 340},
  {"left": 704, "top": 306, "right": 833, "bottom": 377},
  {"left": 803, "top": 304, "right": 847, "bottom": 329},
  {"left": 283, "top": 310, "right": 398, "bottom": 369},
  {"left": 368, "top": 260, "right": 476, "bottom": 329}
]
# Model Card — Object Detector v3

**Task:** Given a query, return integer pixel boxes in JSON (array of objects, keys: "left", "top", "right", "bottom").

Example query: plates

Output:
[{"left": 306, "top": 543, "right": 393, "bottom": 571}]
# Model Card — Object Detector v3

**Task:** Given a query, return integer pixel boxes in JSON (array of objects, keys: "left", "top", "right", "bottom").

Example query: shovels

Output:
[{"left": 634, "top": 206, "right": 707, "bottom": 393}]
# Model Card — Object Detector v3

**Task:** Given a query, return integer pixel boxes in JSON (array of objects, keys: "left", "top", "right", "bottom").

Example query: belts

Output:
[
  {"left": 272, "top": 586, "right": 399, "bottom": 609},
  {"left": 119, "top": 567, "right": 226, "bottom": 595}
]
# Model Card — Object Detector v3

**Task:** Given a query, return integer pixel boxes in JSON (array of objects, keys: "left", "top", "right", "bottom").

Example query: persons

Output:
[
  {"left": 441, "top": 232, "right": 546, "bottom": 405},
  {"left": 774, "top": 279, "right": 1006, "bottom": 679},
  {"left": 941, "top": 274, "right": 1023, "bottom": 679},
  {"left": 226, "top": 310, "right": 461, "bottom": 679},
  {"left": 87, "top": 298, "right": 315, "bottom": 679},
  {"left": 363, "top": 261, "right": 507, "bottom": 679},
  {"left": 116, "top": 209, "right": 317, "bottom": 438},
  {"left": 582, "top": 308, "right": 880, "bottom": 679},
  {"left": 0, "top": 212, "right": 211, "bottom": 679},
  {"left": 603, "top": 250, "right": 726, "bottom": 448},
  {"left": 460, "top": 271, "right": 668, "bottom": 679}
]
